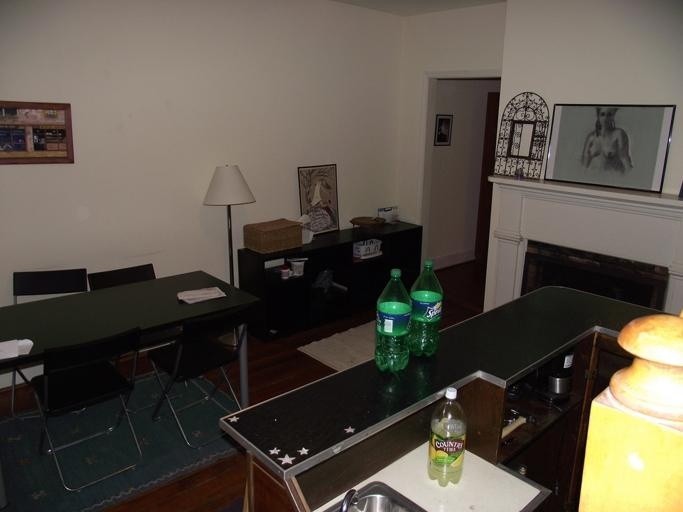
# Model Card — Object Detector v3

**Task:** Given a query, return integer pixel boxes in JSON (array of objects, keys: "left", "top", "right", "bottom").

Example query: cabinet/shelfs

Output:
[{"left": 237, "top": 219, "right": 425, "bottom": 357}]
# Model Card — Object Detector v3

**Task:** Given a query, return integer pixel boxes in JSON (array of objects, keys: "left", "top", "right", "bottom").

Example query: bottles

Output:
[
  {"left": 375, "top": 267, "right": 414, "bottom": 373},
  {"left": 425, "top": 386, "right": 467, "bottom": 489},
  {"left": 410, "top": 259, "right": 445, "bottom": 357}
]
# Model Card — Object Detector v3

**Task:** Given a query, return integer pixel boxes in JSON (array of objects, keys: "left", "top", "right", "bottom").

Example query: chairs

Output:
[
  {"left": 38, "top": 326, "right": 144, "bottom": 492},
  {"left": 149, "top": 309, "right": 242, "bottom": 447},
  {"left": 8, "top": 270, "right": 89, "bottom": 420},
  {"left": 90, "top": 263, "right": 185, "bottom": 418}
]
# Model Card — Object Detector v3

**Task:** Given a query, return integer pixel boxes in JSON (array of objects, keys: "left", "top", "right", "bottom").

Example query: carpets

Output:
[
  {"left": 1, "top": 365, "right": 240, "bottom": 512},
  {"left": 297, "top": 321, "right": 375, "bottom": 369}
]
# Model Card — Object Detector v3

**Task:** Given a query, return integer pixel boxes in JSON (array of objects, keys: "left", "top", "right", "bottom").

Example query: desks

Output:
[{"left": 1, "top": 270, "right": 260, "bottom": 416}]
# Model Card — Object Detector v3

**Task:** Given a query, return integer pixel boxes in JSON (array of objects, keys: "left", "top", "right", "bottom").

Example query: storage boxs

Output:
[{"left": 243, "top": 218, "right": 303, "bottom": 254}]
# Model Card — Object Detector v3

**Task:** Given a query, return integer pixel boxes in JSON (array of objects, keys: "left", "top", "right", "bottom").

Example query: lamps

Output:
[{"left": 203, "top": 164, "right": 257, "bottom": 352}]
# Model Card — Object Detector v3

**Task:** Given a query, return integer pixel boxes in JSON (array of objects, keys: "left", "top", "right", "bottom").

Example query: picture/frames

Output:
[
  {"left": 433, "top": 113, "right": 454, "bottom": 147},
  {"left": 298, "top": 164, "right": 339, "bottom": 234},
  {"left": 0, "top": 100, "right": 75, "bottom": 164},
  {"left": 545, "top": 105, "right": 677, "bottom": 194}
]
